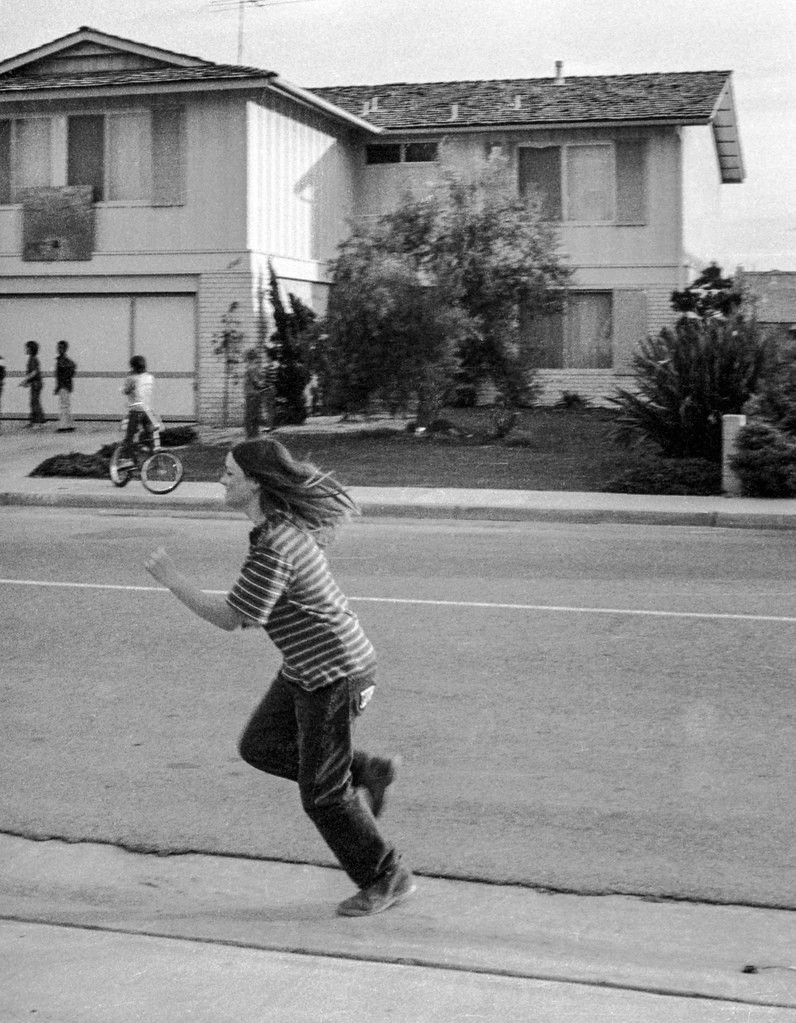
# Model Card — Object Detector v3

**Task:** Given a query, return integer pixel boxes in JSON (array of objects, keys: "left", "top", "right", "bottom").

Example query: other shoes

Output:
[
  {"left": 337, "top": 864, "right": 418, "bottom": 916},
  {"left": 366, "top": 756, "right": 399, "bottom": 818}
]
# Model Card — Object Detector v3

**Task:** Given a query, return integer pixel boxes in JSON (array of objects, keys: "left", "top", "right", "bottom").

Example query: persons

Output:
[
  {"left": 143, "top": 437, "right": 416, "bottom": 915},
  {"left": 52, "top": 340, "right": 77, "bottom": 432},
  {"left": 17, "top": 340, "right": 46, "bottom": 431},
  {"left": 119, "top": 357, "right": 154, "bottom": 450}
]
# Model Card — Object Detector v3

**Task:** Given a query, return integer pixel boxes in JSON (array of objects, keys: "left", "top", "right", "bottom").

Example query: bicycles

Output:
[{"left": 110, "top": 431, "right": 184, "bottom": 494}]
